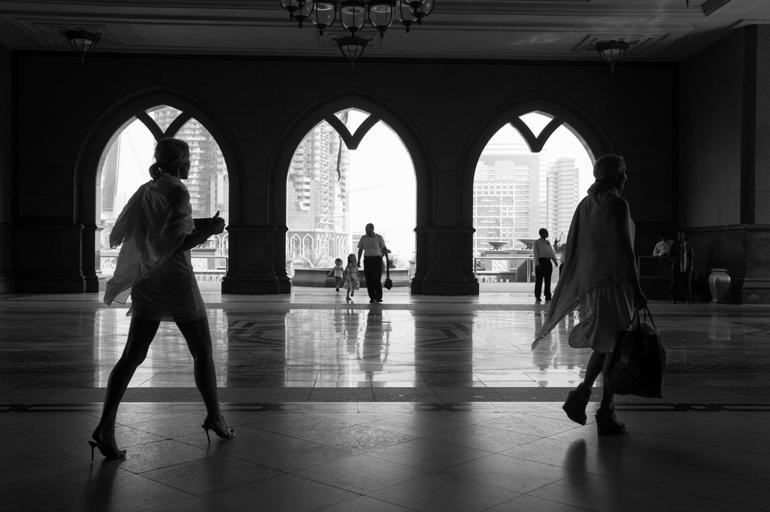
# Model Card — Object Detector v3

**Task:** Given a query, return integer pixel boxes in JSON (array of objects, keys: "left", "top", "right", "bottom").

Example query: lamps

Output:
[
  {"left": 279, "top": 0, "right": 437, "bottom": 69},
  {"left": 65, "top": 31, "right": 101, "bottom": 66},
  {"left": 597, "top": 40, "right": 630, "bottom": 74}
]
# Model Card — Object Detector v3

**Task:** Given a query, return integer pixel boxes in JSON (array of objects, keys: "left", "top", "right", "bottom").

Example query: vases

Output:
[{"left": 708, "top": 268, "right": 731, "bottom": 303}]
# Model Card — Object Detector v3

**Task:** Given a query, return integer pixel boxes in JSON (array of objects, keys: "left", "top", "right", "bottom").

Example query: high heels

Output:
[
  {"left": 88, "top": 425, "right": 127, "bottom": 461},
  {"left": 595, "top": 404, "right": 632, "bottom": 436},
  {"left": 201, "top": 415, "right": 237, "bottom": 442},
  {"left": 562, "top": 384, "right": 592, "bottom": 426}
]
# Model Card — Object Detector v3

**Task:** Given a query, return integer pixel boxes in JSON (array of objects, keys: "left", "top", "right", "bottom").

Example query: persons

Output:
[
  {"left": 356, "top": 224, "right": 389, "bottom": 303},
  {"left": 653, "top": 232, "right": 673, "bottom": 256},
  {"left": 344, "top": 255, "right": 360, "bottom": 301},
  {"left": 89, "top": 137, "right": 237, "bottom": 462},
  {"left": 533, "top": 152, "right": 647, "bottom": 434},
  {"left": 532, "top": 228, "right": 558, "bottom": 301},
  {"left": 669, "top": 231, "right": 696, "bottom": 304},
  {"left": 553, "top": 240, "right": 566, "bottom": 273},
  {"left": 328, "top": 259, "right": 344, "bottom": 296}
]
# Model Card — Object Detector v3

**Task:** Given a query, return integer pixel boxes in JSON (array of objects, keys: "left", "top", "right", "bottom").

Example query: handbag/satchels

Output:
[
  {"left": 603, "top": 305, "right": 665, "bottom": 398},
  {"left": 384, "top": 277, "right": 392, "bottom": 289}
]
